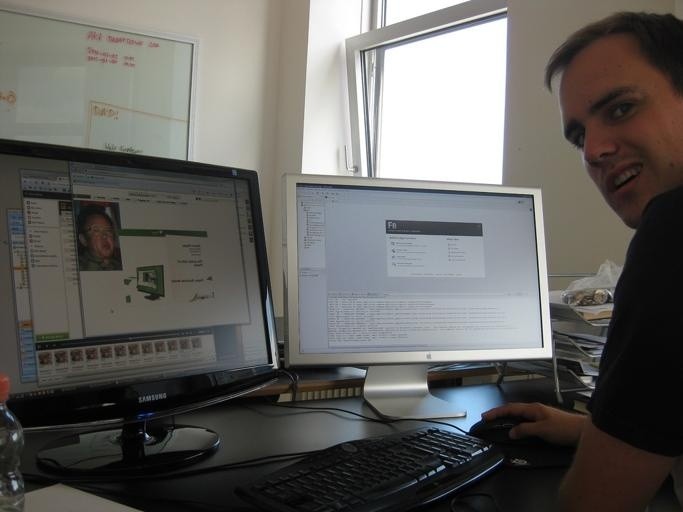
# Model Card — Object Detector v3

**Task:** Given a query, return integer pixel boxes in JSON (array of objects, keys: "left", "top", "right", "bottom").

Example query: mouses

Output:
[{"left": 469, "top": 417, "right": 531, "bottom": 444}]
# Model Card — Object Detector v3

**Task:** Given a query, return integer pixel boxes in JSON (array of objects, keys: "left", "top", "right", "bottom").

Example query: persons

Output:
[
  {"left": 145, "top": 272, "right": 154, "bottom": 282},
  {"left": 481, "top": 10, "right": 683, "bottom": 512},
  {"left": 76, "top": 206, "right": 123, "bottom": 271}
]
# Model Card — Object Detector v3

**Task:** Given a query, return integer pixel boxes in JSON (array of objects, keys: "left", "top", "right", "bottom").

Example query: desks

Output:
[{"left": 18, "top": 316, "right": 651, "bottom": 512}]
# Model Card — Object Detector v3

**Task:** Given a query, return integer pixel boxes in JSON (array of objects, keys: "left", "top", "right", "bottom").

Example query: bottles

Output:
[{"left": 0, "top": 372, "right": 26, "bottom": 511}]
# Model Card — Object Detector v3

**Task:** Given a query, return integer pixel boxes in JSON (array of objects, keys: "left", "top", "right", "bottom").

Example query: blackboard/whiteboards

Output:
[{"left": 1, "top": 5, "right": 200, "bottom": 165}]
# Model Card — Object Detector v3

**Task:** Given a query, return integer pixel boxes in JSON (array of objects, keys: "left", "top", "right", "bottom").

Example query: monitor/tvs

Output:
[
  {"left": 284, "top": 170, "right": 555, "bottom": 419},
  {"left": 0, "top": 135, "right": 282, "bottom": 478}
]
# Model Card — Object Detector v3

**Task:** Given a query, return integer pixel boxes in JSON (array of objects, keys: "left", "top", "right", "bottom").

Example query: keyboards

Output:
[{"left": 233, "top": 425, "right": 506, "bottom": 511}]
